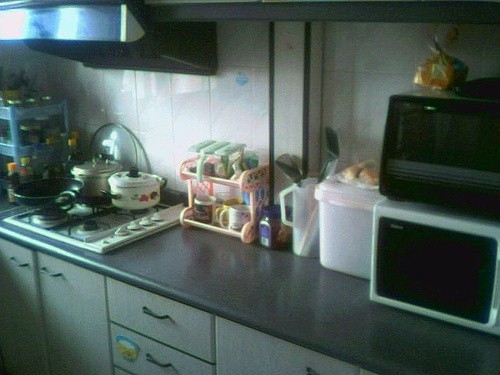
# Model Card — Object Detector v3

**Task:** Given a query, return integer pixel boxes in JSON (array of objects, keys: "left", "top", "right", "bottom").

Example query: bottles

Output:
[
  {"left": 6, "top": 162, "right": 20, "bottom": 205},
  {"left": 19, "top": 156, "right": 33, "bottom": 186},
  {"left": 71, "top": 131, "right": 79, "bottom": 141},
  {"left": 31, "top": 141, "right": 45, "bottom": 178},
  {"left": 59, "top": 133, "right": 68, "bottom": 160},
  {"left": 45, "top": 137, "right": 57, "bottom": 167},
  {"left": 63, "top": 139, "right": 78, "bottom": 174}
]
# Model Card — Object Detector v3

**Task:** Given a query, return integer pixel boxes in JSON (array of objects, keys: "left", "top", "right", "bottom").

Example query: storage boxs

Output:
[{"left": 313, "top": 167, "right": 386, "bottom": 282}]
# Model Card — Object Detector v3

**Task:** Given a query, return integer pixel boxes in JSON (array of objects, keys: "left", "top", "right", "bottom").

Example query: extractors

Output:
[{"left": 0, "top": 0, "right": 217, "bottom": 75}]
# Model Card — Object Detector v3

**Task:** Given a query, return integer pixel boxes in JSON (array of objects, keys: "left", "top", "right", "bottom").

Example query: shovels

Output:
[{"left": 278, "top": 154, "right": 308, "bottom": 230}]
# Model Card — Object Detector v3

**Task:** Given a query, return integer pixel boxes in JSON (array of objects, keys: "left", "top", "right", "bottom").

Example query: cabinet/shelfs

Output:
[
  {"left": 0, "top": 239, "right": 50, "bottom": 374},
  {"left": 105, "top": 276, "right": 216, "bottom": 374},
  {"left": 215, "top": 316, "right": 380, "bottom": 374},
  {"left": 176, "top": 153, "right": 280, "bottom": 244},
  {"left": 1, "top": 94, "right": 72, "bottom": 179},
  {"left": 37, "top": 251, "right": 114, "bottom": 374}
]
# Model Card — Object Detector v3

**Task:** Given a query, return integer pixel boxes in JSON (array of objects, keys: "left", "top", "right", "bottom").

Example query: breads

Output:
[{"left": 413, "top": 55, "right": 469, "bottom": 92}]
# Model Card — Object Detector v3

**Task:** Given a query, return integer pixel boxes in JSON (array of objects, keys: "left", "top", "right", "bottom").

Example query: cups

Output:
[
  {"left": 216, "top": 198, "right": 225, "bottom": 209},
  {"left": 222, "top": 204, "right": 253, "bottom": 229},
  {"left": 216, "top": 198, "right": 241, "bottom": 229},
  {"left": 193, "top": 194, "right": 216, "bottom": 228}
]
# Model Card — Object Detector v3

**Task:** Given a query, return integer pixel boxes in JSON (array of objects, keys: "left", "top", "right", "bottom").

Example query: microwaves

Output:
[
  {"left": 380, "top": 88, "right": 499, "bottom": 211},
  {"left": 368, "top": 199, "right": 499, "bottom": 338}
]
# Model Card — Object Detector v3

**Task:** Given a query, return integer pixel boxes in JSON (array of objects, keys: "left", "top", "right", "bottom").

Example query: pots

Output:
[
  {"left": 108, "top": 167, "right": 163, "bottom": 209},
  {"left": 70, "top": 155, "right": 123, "bottom": 198},
  {"left": 10, "top": 178, "right": 84, "bottom": 211}
]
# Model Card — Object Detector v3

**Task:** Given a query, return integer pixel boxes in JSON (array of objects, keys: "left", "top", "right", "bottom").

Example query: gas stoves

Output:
[{"left": 3, "top": 186, "right": 187, "bottom": 254}]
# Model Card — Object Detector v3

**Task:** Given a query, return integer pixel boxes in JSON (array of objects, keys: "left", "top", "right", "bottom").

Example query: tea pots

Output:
[{"left": 279, "top": 177, "right": 322, "bottom": 258}]
